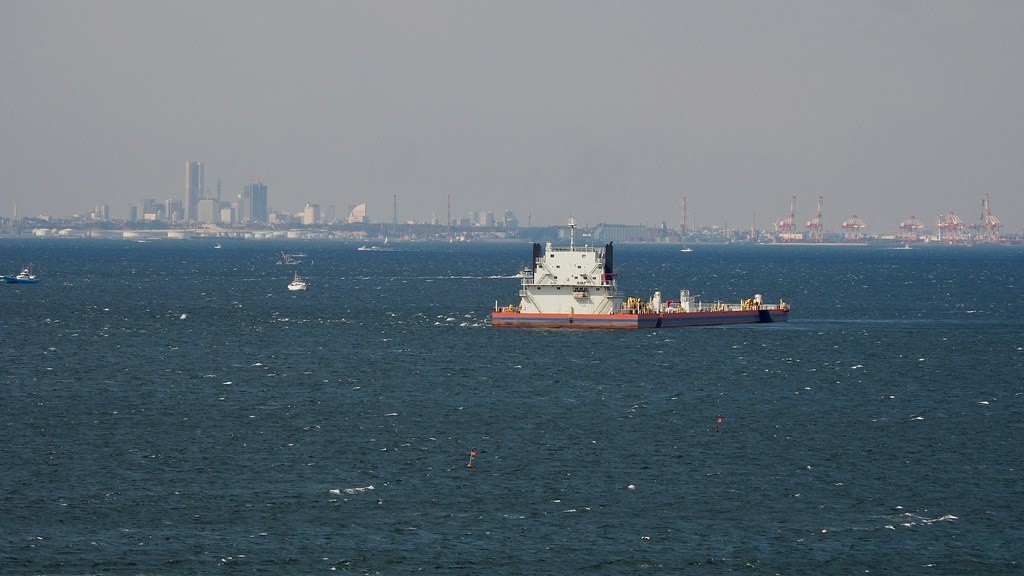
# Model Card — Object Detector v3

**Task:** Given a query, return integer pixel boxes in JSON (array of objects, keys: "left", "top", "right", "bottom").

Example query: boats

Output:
[
  {"left": 287, "top": 275, "right": 310, "bottom": 291},
  {"left": 680, "top": 247, "right": 694, "bottom": 252},
  {"left": 275, "top": 253, "right": 301, "bottom": 265},
  {"left": 357, "top": 245, "right": 380, "bottom": 251},
  {"left": 0, "top": 262, "right": 40, "bottom": 284},
  {"left": 490, "top": 216, "right": 790, "bottom": 329}
]
